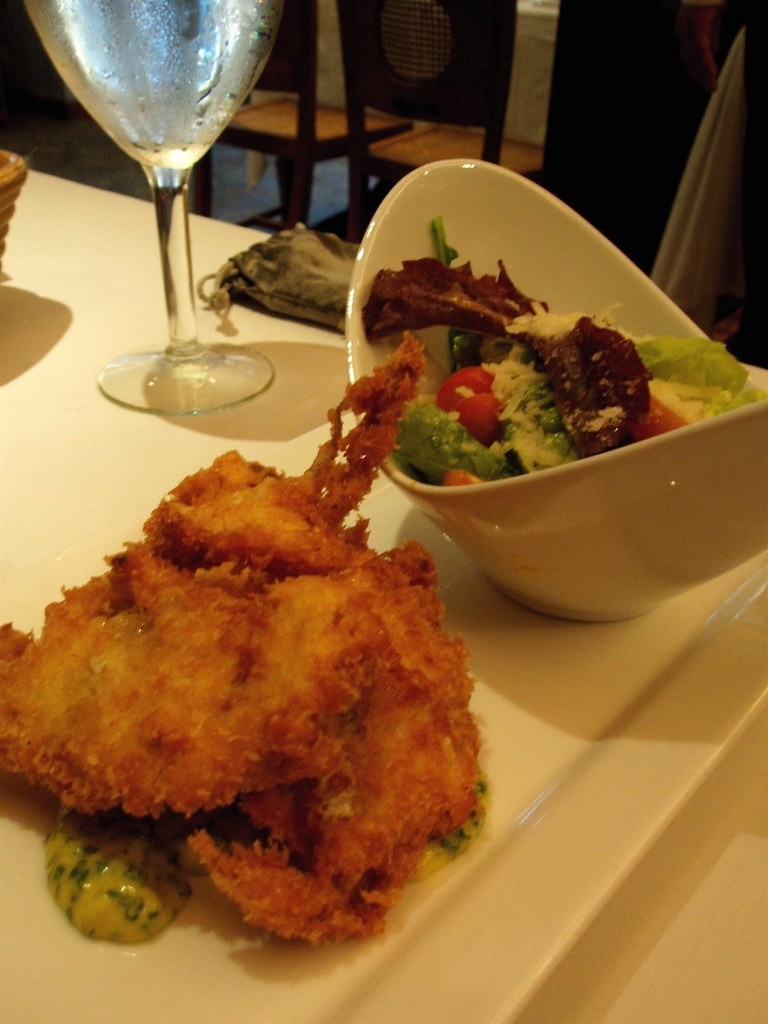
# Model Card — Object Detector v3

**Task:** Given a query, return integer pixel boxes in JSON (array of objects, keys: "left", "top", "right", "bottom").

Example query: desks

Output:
[{"left": 0, "top": 167, "right": 768, "bottom": 1024}]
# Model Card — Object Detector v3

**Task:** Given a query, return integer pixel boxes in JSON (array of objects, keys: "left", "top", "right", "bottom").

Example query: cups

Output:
[{"left": 0, "top": 150, "right": 27, "bottom": 277}]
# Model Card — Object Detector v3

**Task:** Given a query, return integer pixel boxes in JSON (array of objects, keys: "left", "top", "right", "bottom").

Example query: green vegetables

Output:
[{"left": 430, "top": 219, "right": 767, "bottom": 474}]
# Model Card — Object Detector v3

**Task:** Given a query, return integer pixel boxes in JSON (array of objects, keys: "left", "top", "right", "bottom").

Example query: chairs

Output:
[
  {"left": 335, "top": 0, "right": 518, "bottom": 243},
  {"left": 190, "top": 0, "right": 416, "bottom": 232}
]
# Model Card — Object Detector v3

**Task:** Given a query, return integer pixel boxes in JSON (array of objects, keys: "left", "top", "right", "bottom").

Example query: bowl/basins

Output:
[{"left": 343, "top": 155, "right": 768, "bottom": 622}]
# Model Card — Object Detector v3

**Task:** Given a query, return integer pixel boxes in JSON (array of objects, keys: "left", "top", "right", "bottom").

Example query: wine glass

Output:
[{"left": 20, "top": 0, "right": 275, "bottom": 417}]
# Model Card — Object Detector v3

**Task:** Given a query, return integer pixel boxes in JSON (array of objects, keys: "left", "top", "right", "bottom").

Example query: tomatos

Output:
[
  {"left": 631, "top": 395, "right": 687, "bottom": 444},
  {"left": 453, "top": 393, "right": 499, "bottom": 441},
  {"left": 435, "top": 365, "right": 493, "bottom": 413}
]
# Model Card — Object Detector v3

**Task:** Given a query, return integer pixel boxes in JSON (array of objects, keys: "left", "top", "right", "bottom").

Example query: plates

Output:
[{"left": 0, "top": 362, "right": 768, "bottom": 1024}]
